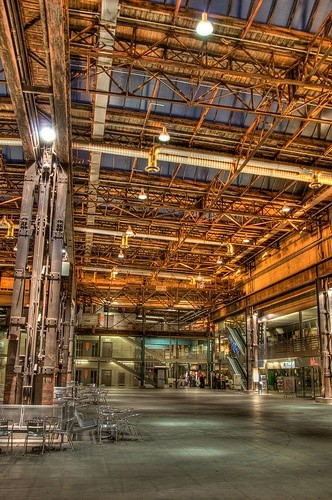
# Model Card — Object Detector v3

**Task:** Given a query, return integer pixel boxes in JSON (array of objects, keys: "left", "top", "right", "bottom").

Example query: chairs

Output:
[{"left": 0, "top": 382, "right": 145, "bottom": 457}]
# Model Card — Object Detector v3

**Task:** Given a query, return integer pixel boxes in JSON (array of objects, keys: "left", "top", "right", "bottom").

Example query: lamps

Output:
[
  {"left": 118, "top": 249, "right": 124, "bottom": 258},
  {"left": 0, "top": 214, "right": 11, "bottom": 229},
  {"left": 13, "top": 243, "right": 18, "bottom": 251},
  {"left": 40, "top": 124, "right": 56, "bottom": 143},
  {"left": 196, "top": 9, "right": 213, "bottom": 37},
  {"left": 217, "top": 256, "right": 222, "bottom": 264},
  {"left": 243, "top": 234, "right": 250, "bottom": 244},
  {"left": 125, "top": 224, "right": 134, "bottom": 234},
  {"left": 281, "top": 202, "right": 290, "bottom": 212},
  {"left": 158, "top": 125, "right": 171, "bottom": 142},
  {"left": 61, "top": 245, "right": 66, "bottom": 254},
  {"left": 198, "top": 272, "right": 202, "bottom": 278},
  {"left": 112, "top": 266, "right": 118, "bottom": 275},
  {"left": 138, "top": 187, "right": 147, "bottom": 200}
]
